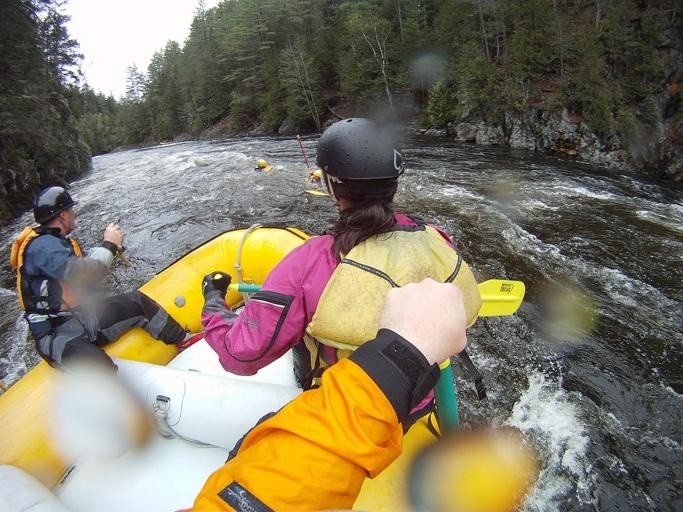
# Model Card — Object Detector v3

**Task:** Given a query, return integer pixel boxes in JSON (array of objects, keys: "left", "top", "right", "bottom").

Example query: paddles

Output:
[
  {"left": 214, "top": 274, "right": 526, "bottom": 316},
  {"left": 305, "top": 189, "right": 329, "bottom": 196}
]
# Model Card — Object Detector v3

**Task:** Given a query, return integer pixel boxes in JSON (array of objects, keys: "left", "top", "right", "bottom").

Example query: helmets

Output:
[
  {"left": 311, "top": 116, "right": 412, "bottom": 181},
  {"left": 31, "top": 185, "right": 81, "bottom": 223}
]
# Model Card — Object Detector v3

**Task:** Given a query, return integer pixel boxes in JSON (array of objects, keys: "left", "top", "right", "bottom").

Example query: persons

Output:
[
  {"left": 311, "top": 170, "right": 322, "bottom": 181},
  {"left": 254, "top": 159, "right": 267, "bottom": 171},
  {"left": 199, "top": 116, "right": 454, "bottom": 436},
  {"left": 190, "top": 278, "right": 467, "bottom": 512},
  {"left": 17, "top": 186, "right": 205, "bottom": 387}
]
